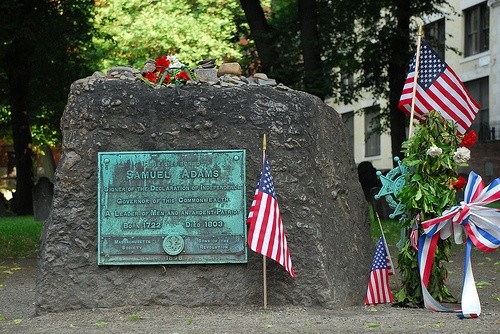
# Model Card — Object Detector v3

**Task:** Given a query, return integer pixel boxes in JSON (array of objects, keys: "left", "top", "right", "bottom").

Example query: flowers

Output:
[
  {"left": 392, "top": 108, "right": 479, "bottom": 309},
  {"left": 143, "top": 55, "right": 197, "bottom": 95}
]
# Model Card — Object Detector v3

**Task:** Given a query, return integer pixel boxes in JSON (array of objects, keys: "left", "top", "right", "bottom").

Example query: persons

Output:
[{"left": 358, "top": 161, "right": 400, "bottom": 222}]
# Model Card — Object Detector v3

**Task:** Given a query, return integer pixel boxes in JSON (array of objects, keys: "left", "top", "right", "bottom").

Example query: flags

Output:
[
  {"left": 246, "top": 152, "right": 298, "bottom": 279},
  {"left": 397, "top": 37, "right": 480, "bottom": 148},
  {"left": 364, "top": 235, "right": 396, "bottom": 306}
]
